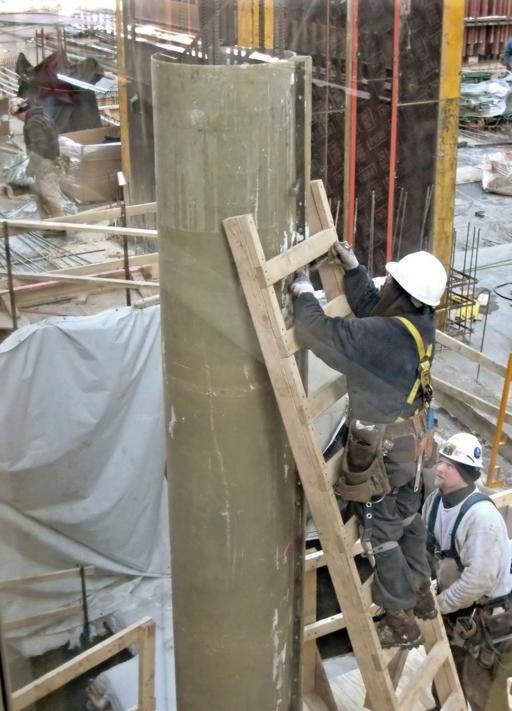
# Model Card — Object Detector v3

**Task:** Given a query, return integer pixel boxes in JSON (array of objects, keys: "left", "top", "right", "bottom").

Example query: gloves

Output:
[
  {"left": 288, "top": 269, "right": 315, "bottom": 298},
  {"left": 328, "top": 240, "right": 360, "bottom": 271},
  {"left": 24, "top": 151, "right": 43, "bottom": 177}
]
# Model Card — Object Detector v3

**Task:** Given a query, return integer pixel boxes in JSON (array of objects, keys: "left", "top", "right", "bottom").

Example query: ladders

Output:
[{"left": 222, "top": 179, "right": 467, "bottom": 711}]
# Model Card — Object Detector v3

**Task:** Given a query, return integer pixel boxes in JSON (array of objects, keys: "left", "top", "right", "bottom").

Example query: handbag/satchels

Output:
[
  {"left": 482, "top": 598, "right": 512, "bottom": 638},
  {"left": 333, "top": 419, "right": 392, "bottom": 505}
]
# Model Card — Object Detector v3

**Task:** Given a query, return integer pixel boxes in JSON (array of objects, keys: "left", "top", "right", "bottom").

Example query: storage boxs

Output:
[
  {"left": 0, "top": 92, "right": 10, "bottom": 136},
  {"left": 58, "top": 125, "right": 122, "bottom": 206}
]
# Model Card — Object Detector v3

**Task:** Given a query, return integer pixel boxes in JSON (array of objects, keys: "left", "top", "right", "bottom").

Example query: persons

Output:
[
  {"left": 8, "top": 97, "right": 67, "bottom": 238},
  {"left": 422, "top": 432, "right": 511, "bottom": 711},
  {"left": 290, "top": 239, "right": 448, "bottom": 650}
]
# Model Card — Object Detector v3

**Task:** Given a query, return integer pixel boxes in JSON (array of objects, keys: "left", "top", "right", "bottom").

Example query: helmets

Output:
[
  {"left": 439, "top": 432, "right": 484, "bottom": 468},
  {"left": 384, "top": 250, "right": 448, "bottom": 307}
]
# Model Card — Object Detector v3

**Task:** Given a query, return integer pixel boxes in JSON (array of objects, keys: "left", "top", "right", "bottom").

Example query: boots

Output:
[{"left": 376, "top": 580, "right": 438, "bottom": 650}]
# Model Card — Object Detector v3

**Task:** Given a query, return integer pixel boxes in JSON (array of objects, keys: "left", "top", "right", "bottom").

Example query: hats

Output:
[{"left": 8, "top": 96, "right": 31, "bottom": 116}]
